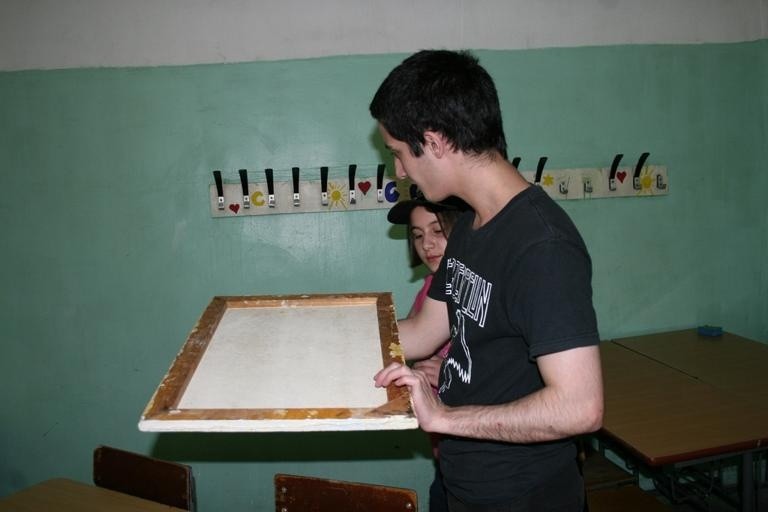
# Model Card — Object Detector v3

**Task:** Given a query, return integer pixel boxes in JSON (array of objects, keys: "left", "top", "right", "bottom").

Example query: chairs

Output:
[
  {"left": 585, "top": 486, "right": 671, "bottom": 512},
  {"left": 93, "top": 446, "right": 192, "bottom": 510},
  {"left": 576, "top": 435, "right": 638, "bottom": 491},
  {"left": 275, "top": 474, "right": 418, "bottom": 512}
]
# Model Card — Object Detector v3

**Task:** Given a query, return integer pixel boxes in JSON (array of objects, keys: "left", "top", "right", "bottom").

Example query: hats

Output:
[{"left": 388, "top": 183, "right": 474, "bottom": 225}]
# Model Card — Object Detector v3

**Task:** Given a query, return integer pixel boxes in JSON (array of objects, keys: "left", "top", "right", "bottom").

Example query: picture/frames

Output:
[{"left": 138, "top": 293, "right": 418, "bottom": 433}]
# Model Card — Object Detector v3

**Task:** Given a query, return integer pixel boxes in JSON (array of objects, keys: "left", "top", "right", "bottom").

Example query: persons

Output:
[
  {"left": 369, "top": 46, "right": 605, "bottom": 512},
  {"left": 398, "top": 183, "right": 466, "bottom": 512}
]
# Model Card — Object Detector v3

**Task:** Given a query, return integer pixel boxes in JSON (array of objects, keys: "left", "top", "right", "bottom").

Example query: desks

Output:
[
  {"left": 1, "top": 479, "right": 190, "bottom": 512},
  {"left": 613, "top": 329, "right": 768, "bottom": 512},
  {"left": 596, "top": 342, "right": 768, "bottom": 512}
]
